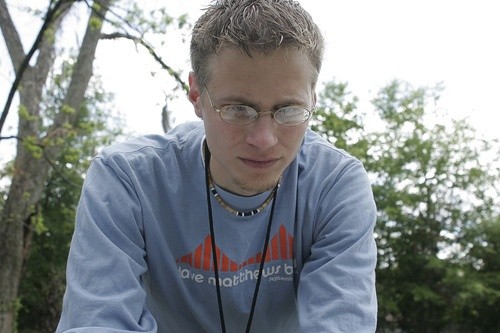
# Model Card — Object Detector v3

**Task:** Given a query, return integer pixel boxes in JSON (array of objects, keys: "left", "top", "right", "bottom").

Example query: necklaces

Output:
[{"left": 203, "top": 146, "right": 282, "bottom": 218}]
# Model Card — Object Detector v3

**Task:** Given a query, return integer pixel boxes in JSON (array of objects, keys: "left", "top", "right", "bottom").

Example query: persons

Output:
[{"left": 55, "top": 0, "right": 377, "bottom": 332}]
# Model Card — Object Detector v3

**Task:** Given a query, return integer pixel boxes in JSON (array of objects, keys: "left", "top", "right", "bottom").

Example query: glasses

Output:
[{"left": 201, "top": 82, "right": 315, "bottom": 126}]
